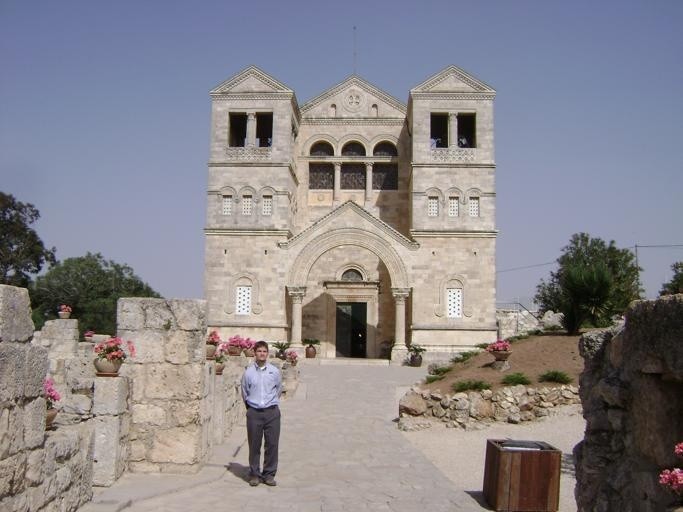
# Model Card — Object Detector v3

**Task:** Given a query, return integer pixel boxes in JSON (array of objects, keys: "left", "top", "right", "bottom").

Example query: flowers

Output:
[
  {"left": 658, "top": 441, "right": 682, "bottom": 504},
  {"left": 486, "top": 339, "right": 511, "bottom": 351},
  {"left": 285, "top": 350, "right": 297, "bottom": 363},
  {"left": 45, "top": 305, "right": 135, "bottom": 410},
  {"left": 206, "top": 331, "right": 256, "bottom": 349}
]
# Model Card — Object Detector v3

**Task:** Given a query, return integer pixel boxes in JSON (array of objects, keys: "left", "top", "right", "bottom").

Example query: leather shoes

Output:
[
  {"left": 263, "top": 474, "right": 276, "bottom": 486},
  {"left": 250, "top": 475, "right": 259, "bottom": 486}
]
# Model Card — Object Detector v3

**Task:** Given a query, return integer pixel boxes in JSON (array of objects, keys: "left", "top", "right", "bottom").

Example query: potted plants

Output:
[
  {"left": 208, "top": 355, "right": 226, "bottom": 374},
  {"left": 273, "top": 341, "right": 288, "bottom": 360},
  {"left": 408, "top": 345, "right": 426, "bottom": 366},
  {"left": 380, "top": 336, "right": 394, "bottom": 359},
  {"left": 302, "top": 338, "right": 319, "bottom": 357}
]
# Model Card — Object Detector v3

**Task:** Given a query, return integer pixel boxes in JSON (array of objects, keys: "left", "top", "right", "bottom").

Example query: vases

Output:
[
  {"left": 46, "top": 407, "right": 57, "bottom": 429},
  {"left": 286, "top": 359, "right": 297, "bottom": 366},
  {"left": 489, "top": 351, "right": 511, "bottom": 360},
  {"left": 206, "top": 344, "right": 255, "bottom": 358}
]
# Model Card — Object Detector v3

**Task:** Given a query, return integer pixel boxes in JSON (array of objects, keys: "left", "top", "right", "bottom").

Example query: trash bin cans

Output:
[{"left": 483, "top": 440, "right": 562, "bottom": 510}]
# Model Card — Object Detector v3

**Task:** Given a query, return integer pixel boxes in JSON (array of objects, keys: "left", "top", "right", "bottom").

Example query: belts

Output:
[{"left": 248, "top": 405, "right": 278, "bottom": 412}]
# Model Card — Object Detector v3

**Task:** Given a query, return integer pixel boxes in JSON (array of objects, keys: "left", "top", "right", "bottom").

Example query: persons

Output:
[{"left": 240, "top": 341, "right": 282, "bottom": 486}]
